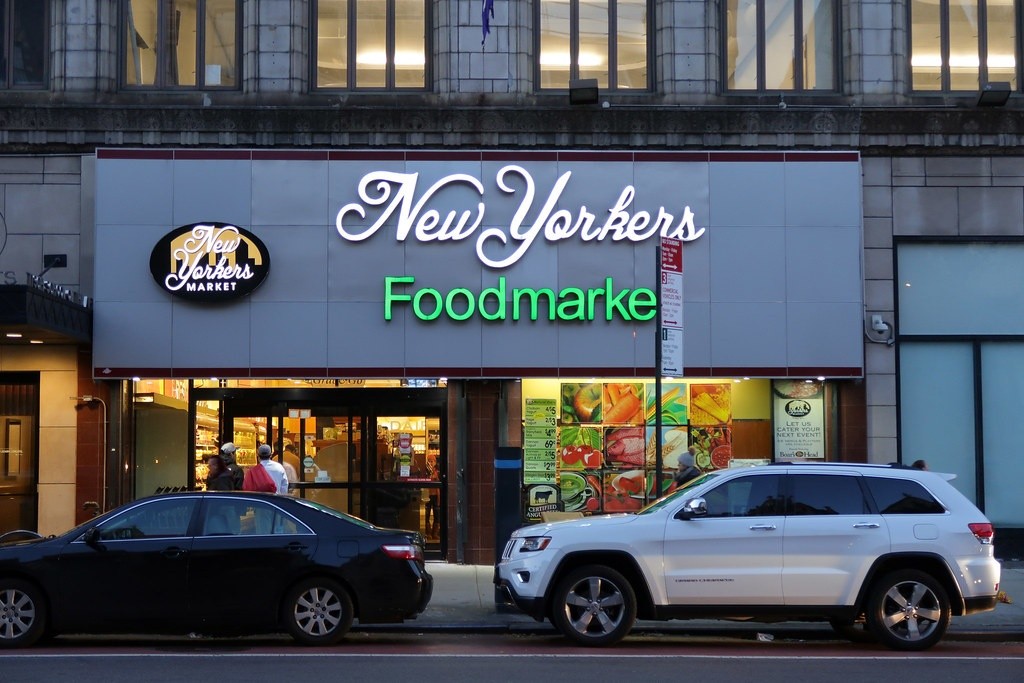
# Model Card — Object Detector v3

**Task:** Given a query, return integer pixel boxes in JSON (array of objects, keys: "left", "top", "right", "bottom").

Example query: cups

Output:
[{"left": 559, "top": 470, "right": 593, "bottom": 502}]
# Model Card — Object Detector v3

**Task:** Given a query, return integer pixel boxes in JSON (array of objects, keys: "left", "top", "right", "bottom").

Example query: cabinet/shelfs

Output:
[{"left": 196, "top": 414, "right": 257, "bottom": 488}]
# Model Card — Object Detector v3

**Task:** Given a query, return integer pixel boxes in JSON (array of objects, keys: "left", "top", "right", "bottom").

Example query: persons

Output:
[
  {"left": 271, "top": 442, "right": 299, "bottom": 480},
  {"left": 422, "top": 463, "right": 439, "bottom": 540},
  {"left": 675, "top": 446, "right": 702, "bottom": 487},
  {"left": 218, "top": 441, "right": 243, "bottom": 489},
  {"left": 243, "top": 442, "right": 289, "bottom": 494},
  {"left": 205, "top": 455, "right": 235, "bottom": 491}
]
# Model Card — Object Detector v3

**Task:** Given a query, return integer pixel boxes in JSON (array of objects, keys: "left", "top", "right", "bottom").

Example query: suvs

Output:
[{"left": 492, "top": 456, "right": 1002, "bottom": 648}]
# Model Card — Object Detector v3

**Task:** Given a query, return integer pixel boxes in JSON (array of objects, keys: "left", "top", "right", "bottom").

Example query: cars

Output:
[{"left": 2, "top": 489, "right": 435, "bottom": 648}]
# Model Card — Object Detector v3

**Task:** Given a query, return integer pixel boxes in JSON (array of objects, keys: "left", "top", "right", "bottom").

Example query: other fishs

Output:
[{"left": 607, "top": 435, "right": 645, "bottom": 457}]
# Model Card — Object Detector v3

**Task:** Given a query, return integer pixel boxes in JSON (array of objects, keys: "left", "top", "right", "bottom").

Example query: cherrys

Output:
[{"left": 560, "top": 428, "right": 600, "bottom": 466}]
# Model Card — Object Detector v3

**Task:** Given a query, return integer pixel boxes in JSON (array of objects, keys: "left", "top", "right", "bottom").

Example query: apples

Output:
[{"left": 562, "top": 385, "right": 602, "bottom": 422}]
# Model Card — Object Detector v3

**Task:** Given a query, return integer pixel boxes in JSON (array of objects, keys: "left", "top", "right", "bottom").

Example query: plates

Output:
[
  {"left": 612, "top": 470, "right": 645, "bottom": 500},
  {"left": 564, "top": 481, "right": 598, "bottom": 513}
]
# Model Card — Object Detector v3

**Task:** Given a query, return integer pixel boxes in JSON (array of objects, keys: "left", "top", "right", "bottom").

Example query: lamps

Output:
[
  {"left": 976, "top": 81, "right": 1011, "bottom": 107},
  {"left": 568, "top": 78, "right": 600, "bottom": 105}
]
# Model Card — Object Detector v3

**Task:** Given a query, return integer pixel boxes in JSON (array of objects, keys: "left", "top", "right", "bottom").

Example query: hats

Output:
[
  {"left": 220, "top": 442, "right": 240, "bottom": 454},
  {"left": 677, "top": 446, "right": 696, "bottom": 467},
  {"left": 257, "top": 444, "right": 271, "bottom": 457}
]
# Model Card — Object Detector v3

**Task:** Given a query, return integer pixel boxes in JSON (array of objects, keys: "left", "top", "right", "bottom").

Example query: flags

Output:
[{"left": 482, "top": 0, "right": 494, "bottom": 37}]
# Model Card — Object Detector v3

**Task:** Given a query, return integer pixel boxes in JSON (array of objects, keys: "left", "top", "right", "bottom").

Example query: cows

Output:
[{"left": 534, "top": 491, "right": 553, "bottom": 504}]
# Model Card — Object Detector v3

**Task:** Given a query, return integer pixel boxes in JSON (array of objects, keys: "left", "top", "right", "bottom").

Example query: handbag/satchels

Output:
[{"left": 242, "top": 464, "right": 276, "bottom": 494}]
